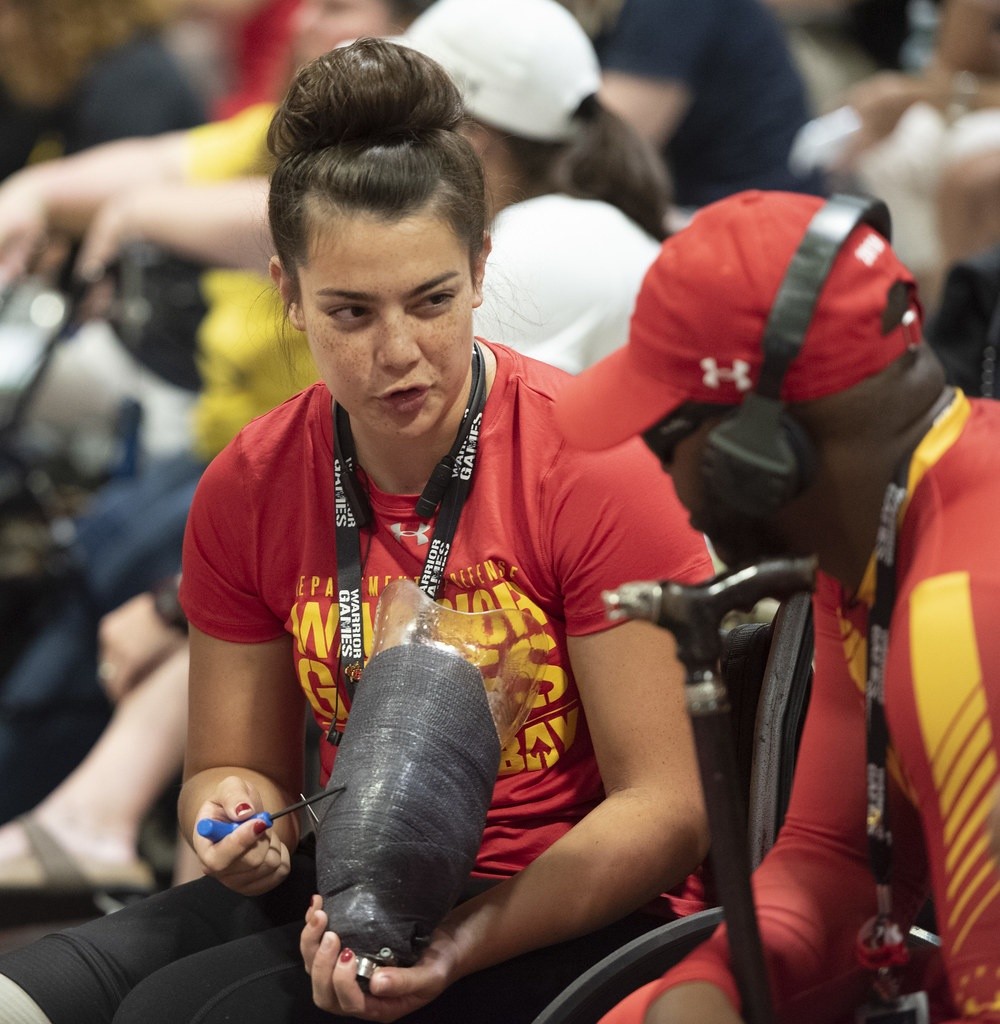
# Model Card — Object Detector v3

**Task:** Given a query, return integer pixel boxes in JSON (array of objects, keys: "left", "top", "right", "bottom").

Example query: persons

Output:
[
  {"left": 0, "top": 39, "right": 736, "bottom": 1021},
  {"left": 0, "top": 0, "right": 1000, "bottom": 904},
  {"left": 547, "top": 191, "right": 999, "bottom": 1024}
]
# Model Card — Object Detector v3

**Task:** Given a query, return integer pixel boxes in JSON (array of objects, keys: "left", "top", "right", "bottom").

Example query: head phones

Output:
[{"left": 702, "top": 191, "right": 897, "bottom": 518}]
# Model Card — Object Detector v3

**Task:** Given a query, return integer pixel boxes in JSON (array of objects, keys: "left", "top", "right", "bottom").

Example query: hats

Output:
[
  {"left": 332, "top": 0, "right": 604, "bottom": 141},
  {"left": 550, "top": 188, "right": 927, "bottom": 453}
]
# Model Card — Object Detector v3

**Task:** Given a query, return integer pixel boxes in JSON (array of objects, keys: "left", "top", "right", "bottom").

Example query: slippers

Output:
[{"left": 0, "top": 813, "right": 154, "bottom": 921}]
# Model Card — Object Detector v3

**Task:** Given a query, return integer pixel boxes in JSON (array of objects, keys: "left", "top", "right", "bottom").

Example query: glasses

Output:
[{"left": 637, "top": 402, "right": 729, "bottom": 473}]
